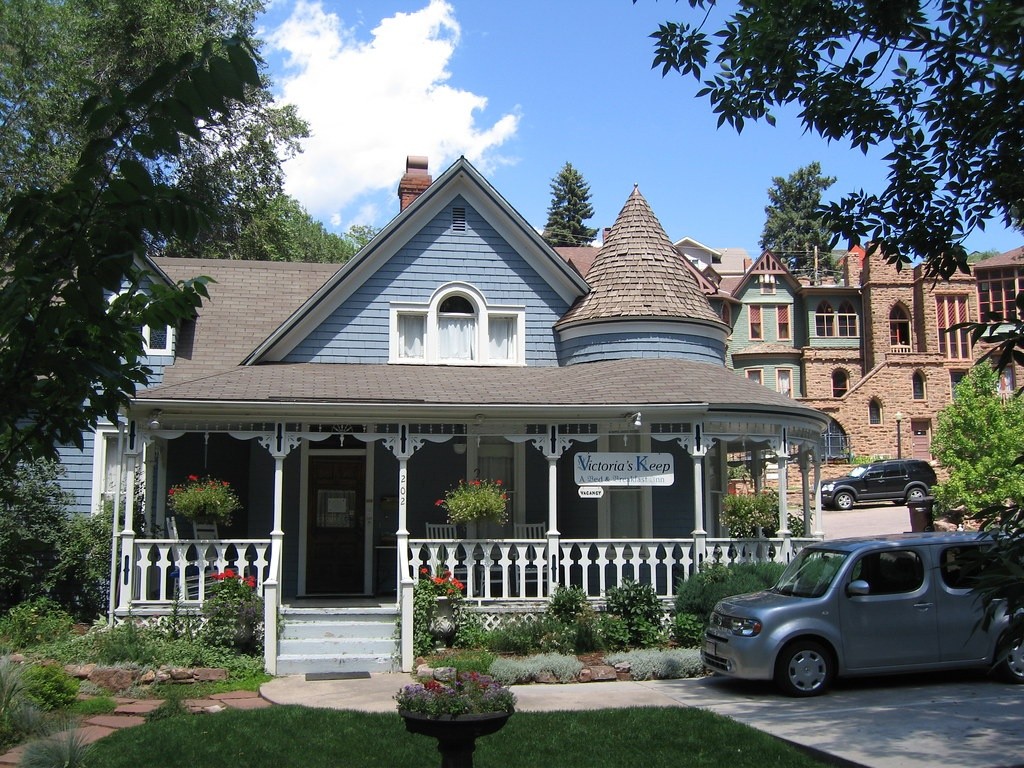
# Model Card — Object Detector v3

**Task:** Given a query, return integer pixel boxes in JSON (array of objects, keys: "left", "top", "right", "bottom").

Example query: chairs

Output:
[
  {"left": 424, "top": 522, "right": 467, "bottom": 599},
  {"left": 513, "top": 522, "right": 554, "bottom": 595},
  {"left": 167, "top": 517, "right": 252, "bottom": 602}
]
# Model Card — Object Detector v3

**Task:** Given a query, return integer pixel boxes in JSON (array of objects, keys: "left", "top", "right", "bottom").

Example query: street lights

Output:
[{"left": 895, "top": 411, "right": 902, "bottom": 459}]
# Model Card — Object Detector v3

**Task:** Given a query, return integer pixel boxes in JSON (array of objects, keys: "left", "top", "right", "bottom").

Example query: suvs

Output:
[{"left": 812, "top": 459, "right": 938, "bottom": 511}]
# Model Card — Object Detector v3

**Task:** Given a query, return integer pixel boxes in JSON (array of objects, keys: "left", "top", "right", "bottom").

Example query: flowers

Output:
[
  {"left": 434, "top": 479, "right": 510, "bottom": 527},
  {"left": 393, "top": 672, "right": 519, "bottom": 718},
  {"left": 421, "top": 569, "right": 465, "bottom": 598},
  {"left": 202, "top": 569, "right": 266, "bottom": 622},
  {"left": 169, "top": 475, "right": 243, "bottom": 529}
]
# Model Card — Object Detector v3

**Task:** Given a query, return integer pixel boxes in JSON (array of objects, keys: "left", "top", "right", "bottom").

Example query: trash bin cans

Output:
[{"left": 907, "top": 496, "right": 935, "bottom": 533}]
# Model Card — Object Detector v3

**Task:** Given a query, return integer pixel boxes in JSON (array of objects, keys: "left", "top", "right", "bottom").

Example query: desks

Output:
[
  {"left": 375, "top": 546, "right": 397, "bottom": 595},
  {"left": 479, "top": 564, "right": 513, "bottom": 598}
]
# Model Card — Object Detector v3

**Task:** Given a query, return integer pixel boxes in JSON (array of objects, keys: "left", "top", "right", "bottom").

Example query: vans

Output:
[{"left": 699, "top": 532, "right": 1024, "bottom": 698}]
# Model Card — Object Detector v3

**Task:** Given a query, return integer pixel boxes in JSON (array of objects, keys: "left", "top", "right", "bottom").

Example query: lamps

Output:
[
  {"left": 148, "top": 409, "right": 164, "bottom": 429},
  {"left": 625, "top": 412, "right": 643, "bottom": 427}
]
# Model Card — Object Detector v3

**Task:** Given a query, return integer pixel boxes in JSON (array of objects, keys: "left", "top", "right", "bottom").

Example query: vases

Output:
[
  {"left": 431, "top": 595, "right": 458, "bottom": 653},
  {"left": 398, "top": 708, "right": 516, "bottom": 768},
  {"left": 232, "top": 618, "right": 252, "bottom": 647}
]
